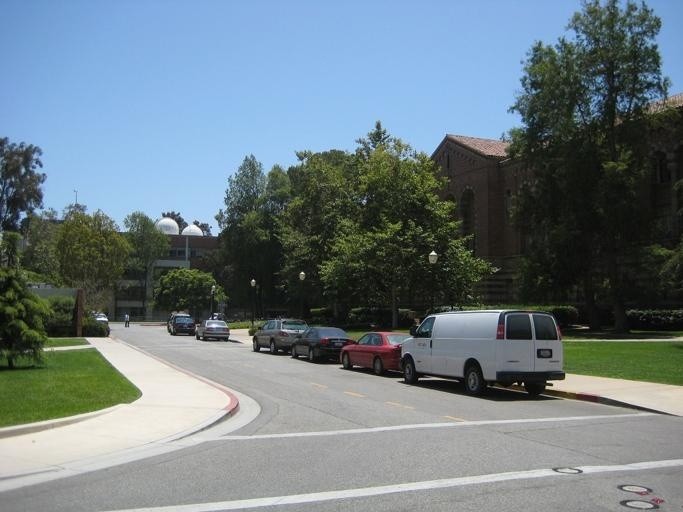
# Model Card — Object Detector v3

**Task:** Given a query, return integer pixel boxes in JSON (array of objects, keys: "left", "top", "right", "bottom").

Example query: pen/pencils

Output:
[{"left": 395, "top": 307, "right": 564, "bottom": 396}]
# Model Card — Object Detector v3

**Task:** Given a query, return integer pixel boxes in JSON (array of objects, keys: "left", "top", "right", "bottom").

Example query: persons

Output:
[
  {"left": 125, "top": 313, "right": 130, "bottom": 327},
  {"left": 410, "top": 319, "right": 421, "bottom": 336}
]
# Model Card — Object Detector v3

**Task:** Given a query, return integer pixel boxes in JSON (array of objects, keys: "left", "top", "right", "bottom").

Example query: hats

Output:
[
  {"left": 166, "top": 311, "right": 231, "bottom": 342},
  {"left": 341, "top": 331, "right": 411, "bottom": 375},
  {"left": 288, "top": 325, "right": 354, "bottom": 364},
  {"left": 94, "top": 313, "right": 109, "bottom": 325}
]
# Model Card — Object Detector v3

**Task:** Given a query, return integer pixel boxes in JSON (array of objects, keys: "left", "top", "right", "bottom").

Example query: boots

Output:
[{"left": 253, "top": 319, "right": 309, "bottom": 354}]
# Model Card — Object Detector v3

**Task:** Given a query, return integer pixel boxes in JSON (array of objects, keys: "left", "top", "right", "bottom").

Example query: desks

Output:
[
  {"left": 209, "top": 282, "right": 215, "bottom": 320},
  {"left": 249, "top": 277, "right": 257, "bottom": 326},
  {"left": 427, "top": 249, "right": 440, "bottom": 313},
  {"left": 298, "top": 269, "right": 306, "bottom": 321}
]
